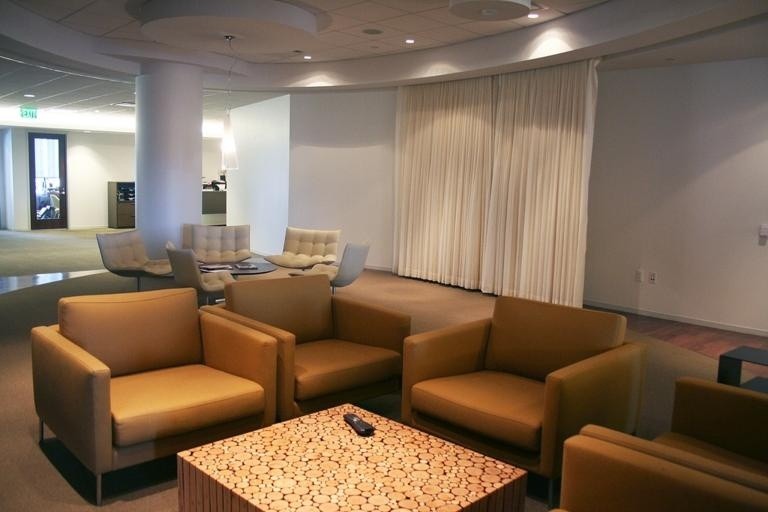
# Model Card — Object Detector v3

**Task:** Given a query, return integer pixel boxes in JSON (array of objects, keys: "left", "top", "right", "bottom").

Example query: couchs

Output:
[
  {"left": 578, "top": 374, "right": 767, "bottom": 493},
  {"left": 551, "top": 437, "right": 767, "bottom": 512},
  {"left": 402, "top": 283, "right": 642, "bottom": 509},
  {"left": 26, "top": 285, "right": 279, "bottom": 506},
  {"left": 205, "top": 272, "right": 412, "bottom": 415}
]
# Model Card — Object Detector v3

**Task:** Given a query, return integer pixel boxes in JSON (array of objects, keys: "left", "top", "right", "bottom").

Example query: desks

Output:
[{"left": 717, "top": 341, "right": 768, "bottom": 391}]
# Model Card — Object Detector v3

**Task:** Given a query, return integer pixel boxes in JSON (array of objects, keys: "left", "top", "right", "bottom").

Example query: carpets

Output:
[{"left": 0, "top": 271, "right": 768, "bottom": 512}]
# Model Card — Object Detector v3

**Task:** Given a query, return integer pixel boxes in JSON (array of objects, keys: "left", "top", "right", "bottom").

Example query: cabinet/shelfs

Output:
[{"left": 107, "top": 181, "right": 136, "bottom": 229}]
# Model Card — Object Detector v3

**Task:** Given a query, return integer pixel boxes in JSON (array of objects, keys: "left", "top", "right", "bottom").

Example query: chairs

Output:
[
  {"left": 306, "top": 243, "right": 370, "bottom": 295},
  {"left": 91, "top": 229, "right": 174, "bottom": 290},
  {"left": 183, "top": 224, "right": 251, "bottom": 264},
  {"left": 161, "top": 238, "right": 233, "bottom": 303},
  {"left": 262, "top": 227, "right": 342, "bottom": 271}
]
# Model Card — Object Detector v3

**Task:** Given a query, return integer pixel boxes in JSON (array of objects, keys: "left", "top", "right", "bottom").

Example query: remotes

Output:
[{"left": 343, "top": 414, "right": 376, "bottom": 436}]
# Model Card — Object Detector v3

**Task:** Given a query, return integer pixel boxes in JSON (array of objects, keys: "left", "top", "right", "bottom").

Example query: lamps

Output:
[{"left": 220, "top": 34, "right": 240, "bottom": 171}]
